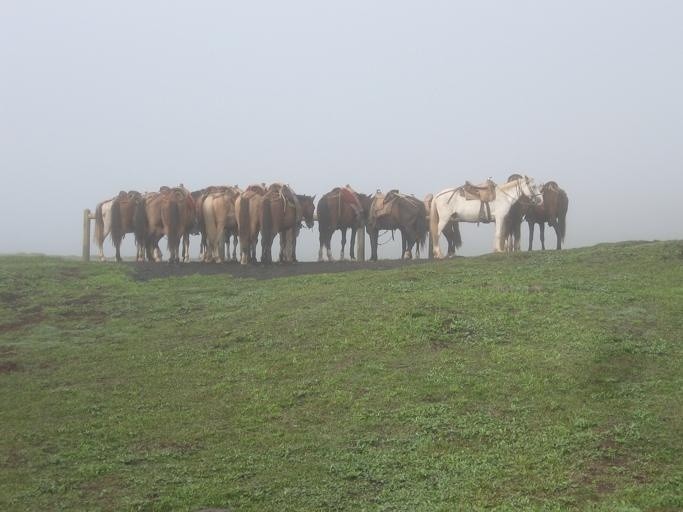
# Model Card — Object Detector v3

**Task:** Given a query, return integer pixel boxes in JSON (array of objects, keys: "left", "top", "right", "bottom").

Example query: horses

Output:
[{"left": 91, "top": 172, "right": 569, "bottom": 266}]
